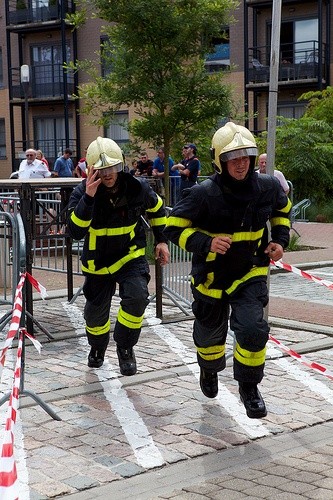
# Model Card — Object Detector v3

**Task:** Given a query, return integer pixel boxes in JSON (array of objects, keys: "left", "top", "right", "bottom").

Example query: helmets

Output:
[
  {"left": 210, "top": 122, "right": 257, "bottom": 176},
  {"left": 86, "top": 137, "right": 125, "bottom": 174}
]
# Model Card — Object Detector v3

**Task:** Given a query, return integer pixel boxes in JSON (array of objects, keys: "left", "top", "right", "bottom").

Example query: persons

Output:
[
  {"left": 122, "top": 154, "right": 138, "bottom": 176},
  {"left": 255, "top": 153, "right": 290, "bottom": 196},
  {"left": 151, "top": 147, "right": 180, "bottom": 207},
  {"left": 66, "top": 136, "right": 169, "bottom": 377},
  {"left": 162, "top": 122, "right": 293, "bottom": 418},
  {"left": 54, "top": 148, "right": 75, "bottom": 178},
  {"left": 171, "top": 144, "right": 201, "bottom": 194},
  {"left": 14, "top": 149, "right": 51, "bottom": 215},
  {"left": 137, "top": 152, "right": 158, "bottom": 194},
  {"left": 76, "top": 147, "right": 87, "bottom": 178},
  {"left": 36, "top": 150, "right": 49, "bottom": 171}
]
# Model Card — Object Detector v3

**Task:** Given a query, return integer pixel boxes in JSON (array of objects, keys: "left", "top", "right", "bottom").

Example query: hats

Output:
[{"left": 184, "top": 144, "right": 195, "bottom": 149}]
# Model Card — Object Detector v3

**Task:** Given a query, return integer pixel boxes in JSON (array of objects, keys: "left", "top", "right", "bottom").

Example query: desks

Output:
[{"left": 279, "top": 68, "right": 296, "bottom": 81}]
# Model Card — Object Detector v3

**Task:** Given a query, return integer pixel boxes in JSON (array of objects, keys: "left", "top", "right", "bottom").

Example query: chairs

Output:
[
  {"left": 298, "top": 52, "right": 318, "bottom": 79},
  {"left": 252, "top": 59, "right": 269, "bottom": 83}
]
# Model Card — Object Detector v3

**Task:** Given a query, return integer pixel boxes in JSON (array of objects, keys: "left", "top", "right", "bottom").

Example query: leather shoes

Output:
[
  {"left": 239, "top": 385, "right": 268, "bottom": 418},
  {"left": 88, "top": 345, "right": 105, "bottom": 368},
  {"left": 117, "top": 344, "right": 137, "bottom": 374},
  {"left": 200, "top": 368, "right": 219, "bottom": 398}
]
